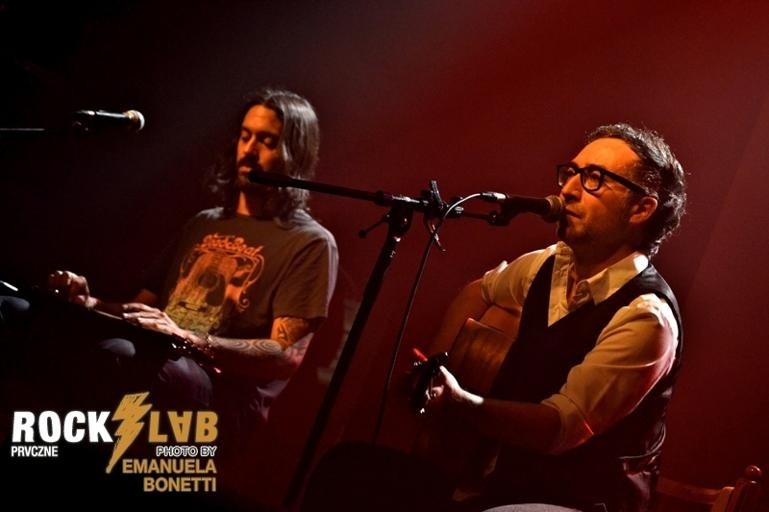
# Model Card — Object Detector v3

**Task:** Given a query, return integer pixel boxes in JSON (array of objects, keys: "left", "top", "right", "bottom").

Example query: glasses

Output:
[{"left": 554, "top": 161, "right": 648, "bottom": 197}]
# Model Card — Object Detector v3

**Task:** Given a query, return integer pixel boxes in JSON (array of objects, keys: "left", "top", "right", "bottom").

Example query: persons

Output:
[
  {"left": 0, "top": 87, "right": 340, "bottom": 511},
  {"left": 302, "top": 124, "right": 688, "bottom": 512}
]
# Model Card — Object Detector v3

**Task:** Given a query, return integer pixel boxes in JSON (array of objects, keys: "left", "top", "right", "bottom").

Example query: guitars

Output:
[{"left": 402, "top": 303, "right": 524, "bottom": 421}]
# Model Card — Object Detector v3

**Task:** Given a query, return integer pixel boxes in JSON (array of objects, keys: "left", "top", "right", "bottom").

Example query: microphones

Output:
[
  {"left": 76, "top": 109, "right": 145, "bottom": 133},
  {"left": 483, "top": 193, "right": 564, "bottom": 223}
]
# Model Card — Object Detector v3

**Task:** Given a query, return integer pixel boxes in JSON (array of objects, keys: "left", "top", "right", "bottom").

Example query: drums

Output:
[{"left": 35, "top": 292, "right": 175, "bottom": 420}]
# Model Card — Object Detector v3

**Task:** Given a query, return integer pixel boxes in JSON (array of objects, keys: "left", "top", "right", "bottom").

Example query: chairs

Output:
[
  {"left": 653, "top": 462, "right": 761, "bottom": 512},
  {"left": 607, "top": 420, "right": 670, "bottom": 511}
]
